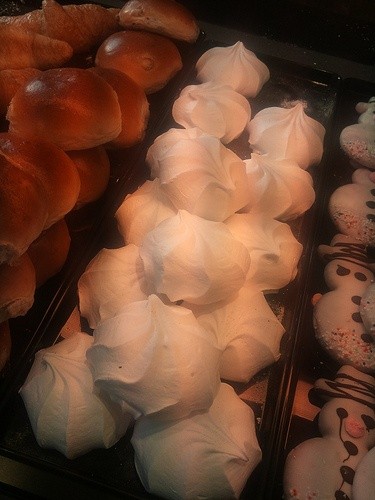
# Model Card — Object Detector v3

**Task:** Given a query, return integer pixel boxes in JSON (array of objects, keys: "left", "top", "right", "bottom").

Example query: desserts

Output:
[{"left": 18, "top": 40, "right": 375, "bottom": 500}]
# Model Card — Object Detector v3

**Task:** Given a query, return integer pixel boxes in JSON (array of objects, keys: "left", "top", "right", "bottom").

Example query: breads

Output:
[{"left": 0, "top": 1, "right": 200, "bottom": 365}]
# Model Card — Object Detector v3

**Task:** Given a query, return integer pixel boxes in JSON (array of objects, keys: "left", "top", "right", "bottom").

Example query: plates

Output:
[{"left": 2, "top": 1, "right": 374, "bottom": 500}]
